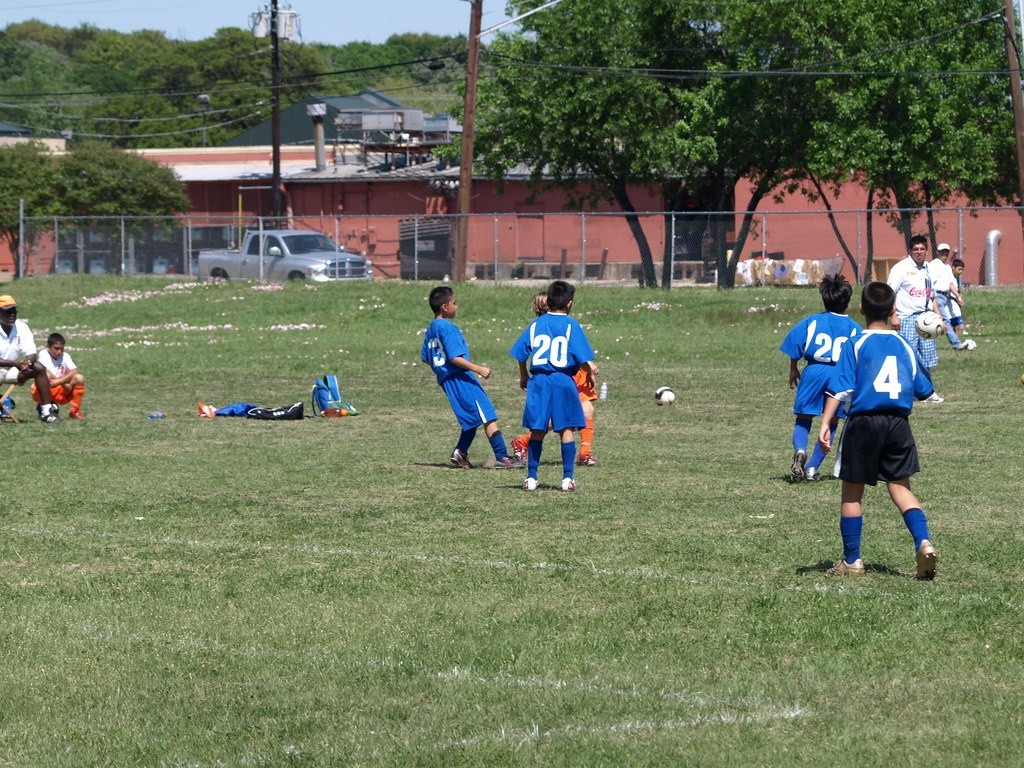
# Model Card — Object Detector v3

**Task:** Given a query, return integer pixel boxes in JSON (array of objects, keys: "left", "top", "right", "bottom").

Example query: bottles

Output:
[
  {"left": 324, "top": 408, "right": 348, "bottom": 417},
  {"left": 2, "top": 396, "right": 11, "bottom": 415},
  {"left": 148, "top": 412, "right": 166, "bottom": 418},
  {"left": 599, "top": 383, "right": 607, "bottom": 399}
]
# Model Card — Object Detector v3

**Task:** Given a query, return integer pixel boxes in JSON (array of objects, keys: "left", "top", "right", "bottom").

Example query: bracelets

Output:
[{"left": 957, "top": 297, "right": 961, "bottom": 300}]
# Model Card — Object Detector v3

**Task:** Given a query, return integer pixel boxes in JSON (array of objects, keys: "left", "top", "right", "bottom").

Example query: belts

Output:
[{"left": 936, "top": 290, "right": 948, "bottom": 297}]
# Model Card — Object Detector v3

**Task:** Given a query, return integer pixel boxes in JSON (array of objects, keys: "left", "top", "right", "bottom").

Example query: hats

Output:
[
  {"left": 937, "top": 243, "right": 950, "bottom": 251},
  {"left": 0, "top": 294, "right": 20, "bottom": 310}
]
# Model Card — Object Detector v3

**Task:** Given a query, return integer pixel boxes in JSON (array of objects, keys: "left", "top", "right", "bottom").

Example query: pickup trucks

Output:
[{"left": 197, "top": 229, "right": 374, "bottom": 284}]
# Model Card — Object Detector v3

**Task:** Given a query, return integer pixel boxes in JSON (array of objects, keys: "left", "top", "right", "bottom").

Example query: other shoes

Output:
[{"left": 40, "top": 414, "right": 58, "bottom": 423}]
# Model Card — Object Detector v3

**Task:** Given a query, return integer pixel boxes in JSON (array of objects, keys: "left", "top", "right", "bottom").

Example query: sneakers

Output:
[
  {"left": 450, "top": 447, "right": 472, "bottom": 468},
  {"left": 576, "top": 453, "right": 599, "bottom": 466},
  {"left": 825, "top": 557, "right": 866, "bottom": 578},
  {"left": 790, "top": 448, "right": 805, "bottom": 481},
  {"left": 921, "top": 391, "right": 943, "bottom": 403},
  {"left": 523, "top": 477, "right": 538, "bottom": 492},
  {"left": 561, "top": 478, "right": 576, "bottom": 492},
  {"left": 70, "top": 410, "right": 85, "bottom": 420},
  {"left": 511, "top": 437, "right": 528, "bottom": 465},
  {"left": 494, "top": 455, "right": 526, "bottom": 469},
  {"left": 805, "top": 466, "right": 819, "bottom": 481},
  {"left": 915, "top": 538, "right": 937, "bottom": 580}
]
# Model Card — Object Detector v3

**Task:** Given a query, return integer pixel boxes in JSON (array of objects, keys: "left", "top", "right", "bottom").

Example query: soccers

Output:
[
  {"left": 915, "top": 310, "right": 945, "bottom": 340},
  {"left": 654, "top": 386, "right": 676, "bottom": 406},
  {"left": 965, "top": 339, "right": 977, "bottom": 351}
]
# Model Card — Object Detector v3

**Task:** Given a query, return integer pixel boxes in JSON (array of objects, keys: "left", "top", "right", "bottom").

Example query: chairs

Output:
[{"left": 0, "top": 379, "right": 21, "bottom": 426}]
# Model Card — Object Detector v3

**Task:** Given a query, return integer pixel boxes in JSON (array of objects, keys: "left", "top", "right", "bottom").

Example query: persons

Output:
[
  {"left": 780, "top": 274, "right": 862, "bottom": 482},
  {"left": 510, "top": 292, "right": 600, "bottom": 466},
  {"left": 818, "top": 282, "right": 938, "bottom": 582},
  {"left": 0, "top": 294, "right": 63, "bottom": 423},
  {"left": 929, "top": 243, "right": 969, "bottom": 351},
  {"left": 947, "top": 259, "right": 965, "bottom": 337},
  {"left": 887, "top": 234, "right": 944, "bottom": 403},
  {"left": 420, "top": 287, "right": 526, "bottom": 470},
  {"left": 509, "top": 281, "right": 595, "bottom": 492},
  {"left": 32, "top": 333, "right": 85, "bottom": 418}
]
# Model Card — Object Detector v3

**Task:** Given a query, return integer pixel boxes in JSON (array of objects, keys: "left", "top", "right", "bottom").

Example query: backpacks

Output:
[
  {"left": 246, "top": 403, "right": 304, "bottom": 420},
  {"left": 312, "top": 375, "right": 358, "bottom": 417}
]
